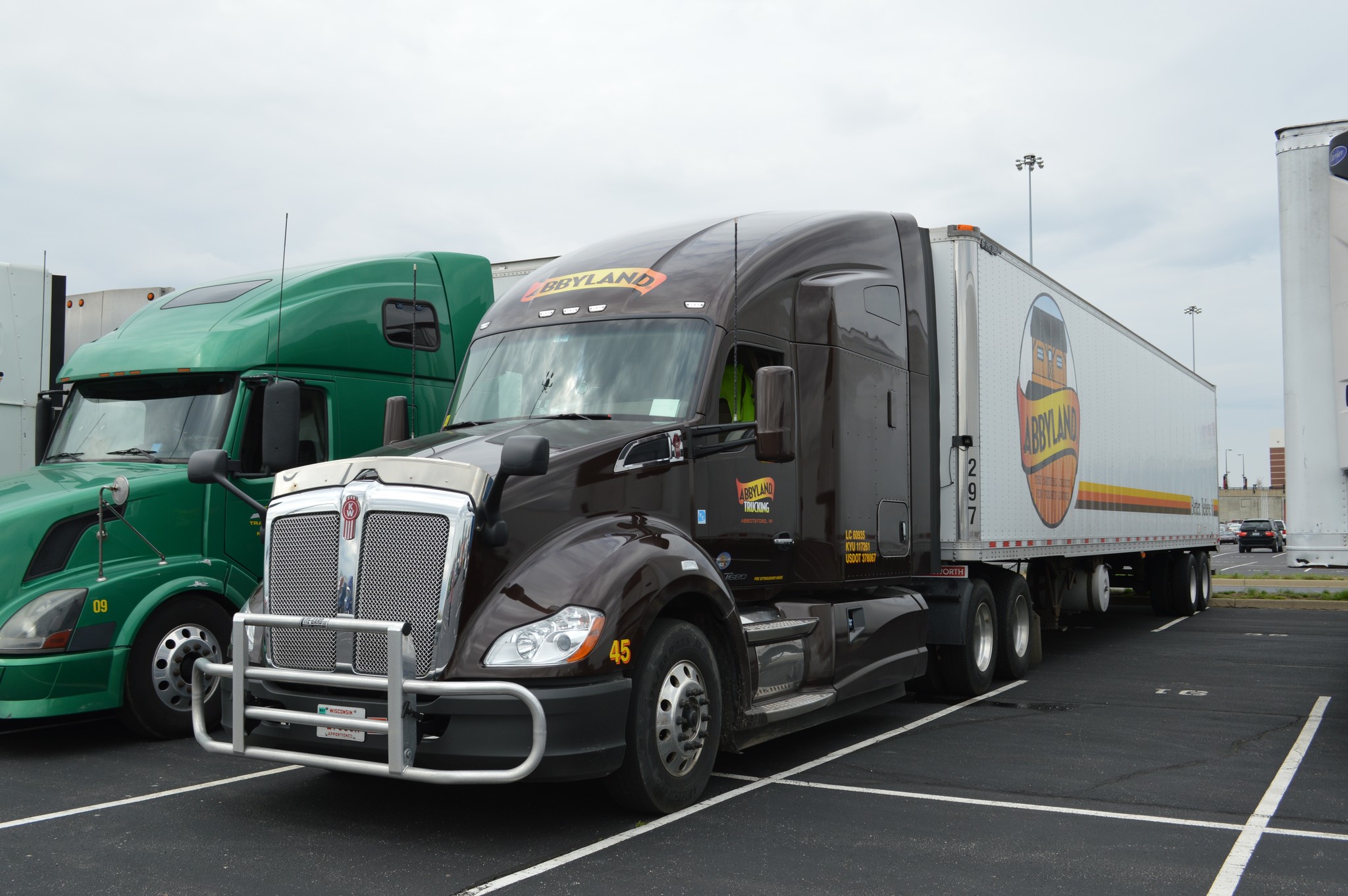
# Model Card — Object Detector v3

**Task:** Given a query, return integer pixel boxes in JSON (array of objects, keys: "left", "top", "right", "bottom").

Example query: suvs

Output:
[
  {"left": 1273, "top": 519, "right": 1286, "bottom": 546},
  {"left": 1237, "top": 518, "right": 1285, "bottom": 553}
]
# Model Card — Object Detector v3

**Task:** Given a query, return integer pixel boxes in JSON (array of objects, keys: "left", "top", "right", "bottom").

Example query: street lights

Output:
[
  {"left": 1015, "top": 152, "right": 1046, "bottom": 266},
  {"left": 1183, "top": 304, "right": 1203, "bottom": 373}
]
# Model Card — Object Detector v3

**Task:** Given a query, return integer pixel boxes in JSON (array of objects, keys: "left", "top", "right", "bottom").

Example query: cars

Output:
[
  {"left": 1219, "top": 530, "right": 1238, "bottom": 545},
  {"left": 1220, "top": 519, "right": 1244, "bottom": 537}
]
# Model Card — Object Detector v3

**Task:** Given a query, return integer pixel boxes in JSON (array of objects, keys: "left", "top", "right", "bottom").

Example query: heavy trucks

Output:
[
  {"left": 189, "top": 204, "right": 1224, "bottom": 820},
  {"left": 2, "top": 249, "right": 559, "bottom": 747}
]
